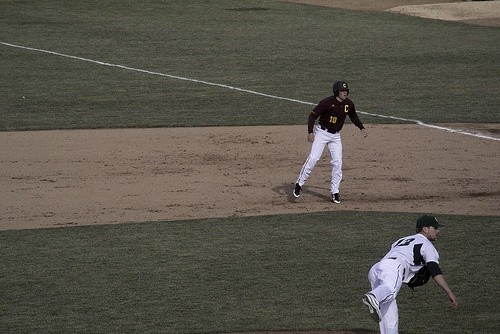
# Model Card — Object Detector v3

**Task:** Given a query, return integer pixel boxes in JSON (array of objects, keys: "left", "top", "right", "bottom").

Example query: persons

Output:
[
  {"left": 362, "top": 214, "right": 458, "bottom": 334},
  {"left": 293, "top": 81, "right": 368, "bottom": 204}
]
